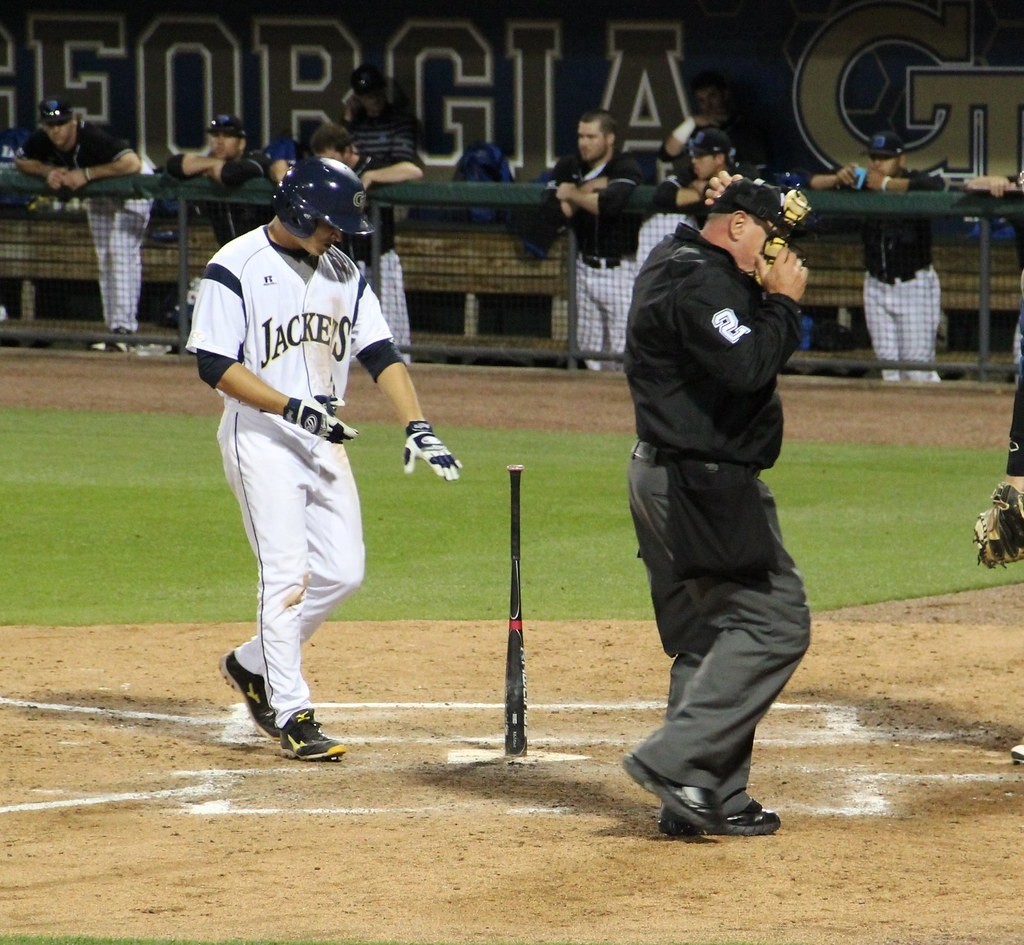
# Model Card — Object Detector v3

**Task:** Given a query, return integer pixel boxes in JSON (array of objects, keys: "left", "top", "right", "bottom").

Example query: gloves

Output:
[
  {"left": 283, "top": 394, "right": 360, "bottom": 445},
  {"left": 403, "top": 420, "right": 463, "bottom": 482}
]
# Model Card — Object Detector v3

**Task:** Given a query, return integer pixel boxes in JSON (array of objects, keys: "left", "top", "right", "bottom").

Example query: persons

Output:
[
  {"left": 184, "top": 157, "right": 462, "bottom": 760},
  {"left": 0, "top": 66, "right": 424, "bottom": 366},
  {"left": 624, "top": 171, "right": 810, "bottom": 837},
  {"left": 550, "top": 74, "right": 1024, "bottom": 764}
]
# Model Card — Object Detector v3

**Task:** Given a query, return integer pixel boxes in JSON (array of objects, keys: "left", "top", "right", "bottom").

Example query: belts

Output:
[
  {"left": 630, "top": 440, "right": 763, "bottom": 480},
  {"left": 582, "top": 254, "right": 623, "bottom": 268},
  {"left": 860, "top": 256, "right": 933, "bottom": 285}
]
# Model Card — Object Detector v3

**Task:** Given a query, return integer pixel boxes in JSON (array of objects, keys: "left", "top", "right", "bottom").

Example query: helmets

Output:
[
  {"left": 39, "top": 97, "right": 74, "bottom": 125},
  {"left": 273, "top": 156, "right": 376, "bottom": 238},
  {"left": 353, "top": 65, "right": 390, "bottom": 98},
  {"left": 202, "top": 112, "right": 246, "bottom": 139}
]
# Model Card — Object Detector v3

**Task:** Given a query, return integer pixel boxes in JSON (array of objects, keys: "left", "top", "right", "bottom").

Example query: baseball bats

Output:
[{"left": 503, "top": 463, "right": 528, "bottom": 757}]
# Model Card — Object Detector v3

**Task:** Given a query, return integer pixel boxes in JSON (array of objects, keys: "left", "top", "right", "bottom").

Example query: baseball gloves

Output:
[{"left": 975, "top": 485, "right": 1023, "bottom": 568}]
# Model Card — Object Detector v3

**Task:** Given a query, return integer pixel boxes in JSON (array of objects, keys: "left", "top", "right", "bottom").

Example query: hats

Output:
[
  {"left": 713, "top": 178, "right": 808, "bottom": 240},
  {"left": 686, "top": 128, "right": 731, "bottom": 153},
  {"left": 859, "top": 131, "right": 905, "bottom": 157}
]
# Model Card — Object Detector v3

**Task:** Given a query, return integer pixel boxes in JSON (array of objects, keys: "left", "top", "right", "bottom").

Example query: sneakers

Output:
[
  {"left": 89, "top": 328, "right": 131, "bottom": 352},
  {"left": 279, "top": 707, "right": 346, "bottom": 761},
  {"left": 658, "top": 795, "right": 782, "bottom": 837},
  {"left": 623, "top": 752, "right": 725, "bottom": 833},
  {"left": 219, "top": 650, "right": 282, "bottom": 741}
]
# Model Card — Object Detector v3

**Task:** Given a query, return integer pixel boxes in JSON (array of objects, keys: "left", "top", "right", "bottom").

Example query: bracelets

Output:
[
  {"left": 84, "top": 167, "right": 91, "bottom": 182},
  {"left": 882, "top": 176, "right": 891, "bottom": 192}
]
